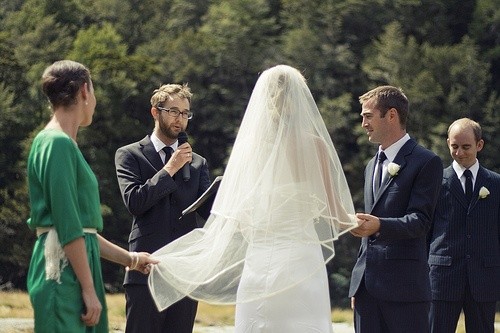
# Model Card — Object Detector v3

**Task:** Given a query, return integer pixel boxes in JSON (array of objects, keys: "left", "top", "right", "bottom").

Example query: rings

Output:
[{"left": 187, "top": 153, "right": 190, "bottom": 157}]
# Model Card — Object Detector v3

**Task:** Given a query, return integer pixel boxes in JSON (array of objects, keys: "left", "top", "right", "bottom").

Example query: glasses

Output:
[{"left": 157, "top": 107, "right": 193, "bottom": 119}]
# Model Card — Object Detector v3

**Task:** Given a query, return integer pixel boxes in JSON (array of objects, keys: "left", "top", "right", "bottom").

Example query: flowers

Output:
[
  {"left": 479, "top": 186, "right": 492, "bottom": 198},
  {"left": 388, "top": 162, "right": 402, "bottom": 176}
]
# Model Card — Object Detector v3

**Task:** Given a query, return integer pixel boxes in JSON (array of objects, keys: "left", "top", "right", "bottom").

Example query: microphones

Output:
[{"left": 178, "top": 131, "right": 190, "bottom": 182}]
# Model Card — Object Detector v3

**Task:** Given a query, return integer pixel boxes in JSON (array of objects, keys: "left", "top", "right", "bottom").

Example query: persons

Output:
[
  {"left": 148, "top": 64, "right": 444, "bottom": 333},
  {"left": 114, "top": 83, "right": 220, "bottom": 333},
  {"left": 428, "top": 118, "right": 500, "bottom": 333},
  {"left": 25, "top": 58, "right": 161, "bottom": 333}
]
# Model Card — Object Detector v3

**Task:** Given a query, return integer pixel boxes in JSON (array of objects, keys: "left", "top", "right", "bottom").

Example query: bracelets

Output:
[{"left": 130, "top": 250, "right": 139, "bottom": 270}]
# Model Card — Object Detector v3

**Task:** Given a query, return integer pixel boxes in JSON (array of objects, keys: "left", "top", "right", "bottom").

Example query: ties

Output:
[
  {"left": 162, "top": 147, "right": 175, "bottom": 181},
  {"left": 464, "top": 170, "right": 474, "bottom": 204},
  {"left": 374, "top": 151, "right": 387, "bottom": 198}
]
